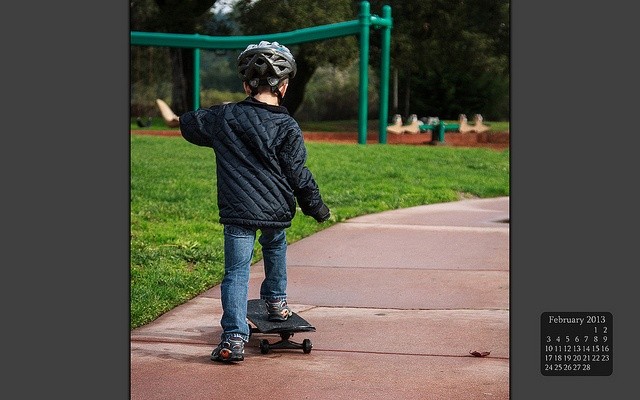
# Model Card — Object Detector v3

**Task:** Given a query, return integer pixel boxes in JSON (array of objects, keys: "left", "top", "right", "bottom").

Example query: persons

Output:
[{"left": 155, "top": 40, "right": 331, "bottom": 362}]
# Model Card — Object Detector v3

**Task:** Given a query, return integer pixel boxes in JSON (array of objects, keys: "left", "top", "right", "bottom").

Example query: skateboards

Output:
[{"left": 247, "top": 299, "right": 316, "bottom": 353}]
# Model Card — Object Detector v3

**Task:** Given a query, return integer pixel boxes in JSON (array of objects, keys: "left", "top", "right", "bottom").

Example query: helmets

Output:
[{"left": 237, "top": 40, "right": 297, "bottom": 89}]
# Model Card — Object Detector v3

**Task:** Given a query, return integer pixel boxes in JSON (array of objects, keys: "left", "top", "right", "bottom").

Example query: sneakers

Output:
[
  {"left": 265, "top": 299, "right": 293, "bottom": 321},
  {"left": 211, "top": 336, "right": 246, "bottom": 362}
]
